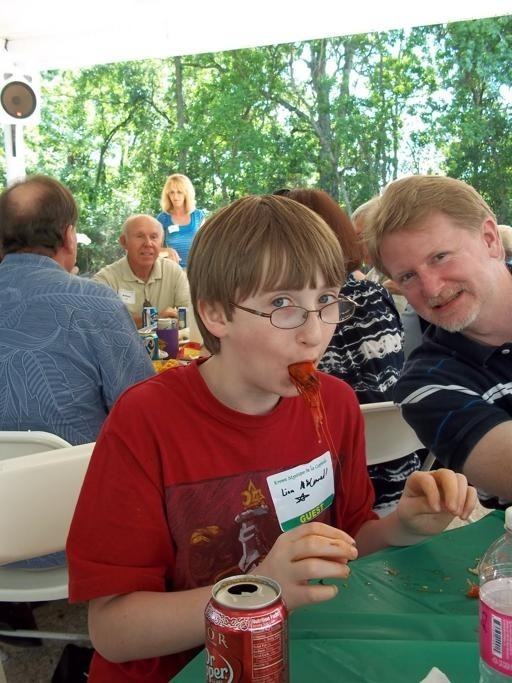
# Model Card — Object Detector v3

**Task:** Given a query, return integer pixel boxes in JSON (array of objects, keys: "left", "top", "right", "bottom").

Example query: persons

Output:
[
  {"left": 283, "top": 186, "right": 433, "bottom": 519},
  {"left": 157, "top": 172, "right": 206, "bottom": 268},
  {"left": 91, "top": 212, "right": 193, "bottom": 331},
  {"left": 399, "top": 300, "right": 435, "bottom": 361},
  {"left": 61, "top": 194, "right": 481, "bottom": 682},
  {"left": 365, "top": 176, "right": 512, "bottom": 511},
  {"left": 496, "top": 223, "right": 512, "bottom": 270},
  {"left": 347, "top": 194, "right": 402, "bottom": 295},
  {"left": 1, "top": 174, "right": 157, "bottom": 571}
]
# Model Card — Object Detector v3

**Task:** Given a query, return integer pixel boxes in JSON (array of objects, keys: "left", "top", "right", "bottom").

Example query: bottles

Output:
[{"left": 474, "top": 503, "right": 512, "bottom": 683}]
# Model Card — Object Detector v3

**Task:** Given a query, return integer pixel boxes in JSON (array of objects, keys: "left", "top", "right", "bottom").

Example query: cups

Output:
[{"left": 157, "top": 317, "right": 178, "bottom": 359}]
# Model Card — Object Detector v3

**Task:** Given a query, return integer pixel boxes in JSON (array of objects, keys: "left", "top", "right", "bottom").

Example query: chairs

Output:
[{"left": 0, "top": 399, "right": 439, "bottom": 682}]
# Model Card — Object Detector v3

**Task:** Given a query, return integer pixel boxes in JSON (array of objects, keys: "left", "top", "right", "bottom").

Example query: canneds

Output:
[
  {"left": 138, "top": 329, "right": 159, "bottom": 360},
  {"left": 142, "top": 306, "right": 158, "bottom": 331},
  {"left": 202, "top": 574, "right": 289, "bottom": 683}
]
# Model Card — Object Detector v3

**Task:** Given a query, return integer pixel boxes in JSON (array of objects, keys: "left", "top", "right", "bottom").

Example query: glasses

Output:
[{"left": 224, "top": 294, "right": 355, "bottom": 330}]
